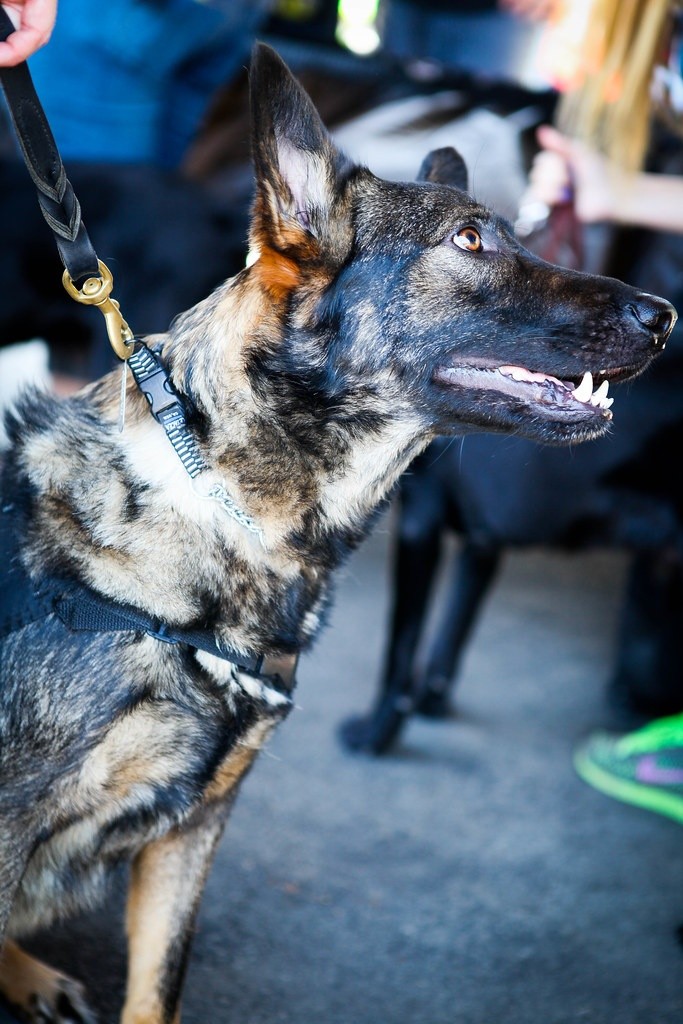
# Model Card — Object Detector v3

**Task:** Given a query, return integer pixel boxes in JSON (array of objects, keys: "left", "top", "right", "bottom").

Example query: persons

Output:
[
  {"left": 0, "top": 0, "right": 284, "bottom": 348},
  {"left": 536, "top": 125, "right": 683, "bottom": 235}
]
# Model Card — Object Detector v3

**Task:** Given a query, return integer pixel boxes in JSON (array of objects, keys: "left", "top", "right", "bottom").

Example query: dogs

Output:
[{"left": 0, "top": 41, "right": 679, "bottom": 1024}]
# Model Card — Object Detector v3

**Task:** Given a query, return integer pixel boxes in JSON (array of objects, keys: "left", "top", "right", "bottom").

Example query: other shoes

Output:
[
  {"left": 338, "top": 714, "right": 397, "bottom": 758},
  {"left": 417, "top": 678, "right": 449, "bottom": 721},
  {"left": 577, "top": 710, "right": 682, "bottom": 819}
]
